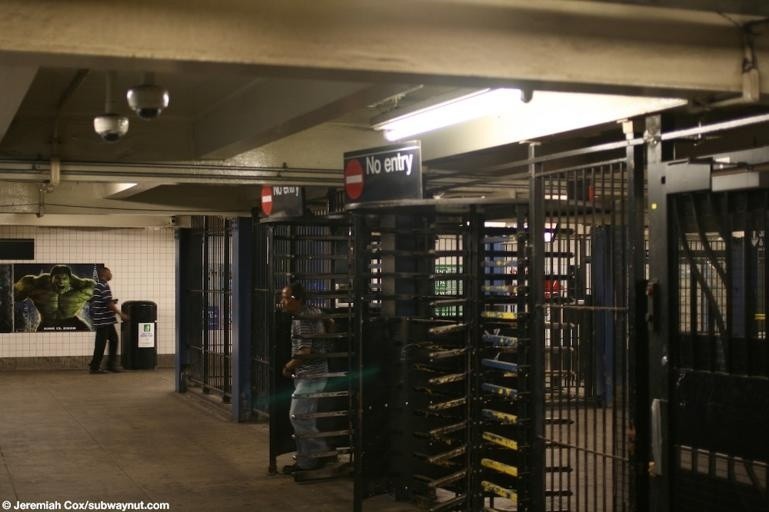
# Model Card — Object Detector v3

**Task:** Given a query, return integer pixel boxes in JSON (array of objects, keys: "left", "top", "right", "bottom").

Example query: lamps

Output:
[
  {"left": 93, "top": 70, "right": 131, "bottom": 140},
  {"left": 370, "top": 87, "right": 525, "bottom": 142},
  {"left": 125, "top": 70, "right": 171, "bottom": 121}
]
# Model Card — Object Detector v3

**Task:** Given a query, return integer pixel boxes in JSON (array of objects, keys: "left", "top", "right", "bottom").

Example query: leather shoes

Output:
[{"left": 283, "top": 463, "right": 304, "bottom": 473}]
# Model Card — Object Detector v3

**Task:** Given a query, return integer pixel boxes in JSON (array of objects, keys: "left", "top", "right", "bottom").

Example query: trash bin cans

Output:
[{"left": 121, "top": 301, "right": 157, "bottom": 370}]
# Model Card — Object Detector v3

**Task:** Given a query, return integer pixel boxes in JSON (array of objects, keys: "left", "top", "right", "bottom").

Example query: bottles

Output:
[{"left": 753, "top": 314, "right": 766, "bottom": 340}]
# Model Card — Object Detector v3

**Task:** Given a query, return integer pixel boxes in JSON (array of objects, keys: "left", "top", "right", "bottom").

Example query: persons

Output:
[
  {"left": 279, "top": 282, "right": 336, "bottom": 475},
  {"left": 88, "top": 267, "right": 129, "bottom": 374},
  {"left": 14, "top": 265, "right": 97, "bottom": 330}
]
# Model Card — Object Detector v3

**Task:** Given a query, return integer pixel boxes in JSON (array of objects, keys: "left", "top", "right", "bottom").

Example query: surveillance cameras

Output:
[
  {"left": 93, "top": 114, "right": 129, "bottom": 141},
  {"left": 127, "top": 84, "right": 170, "bottom": 120}
]
